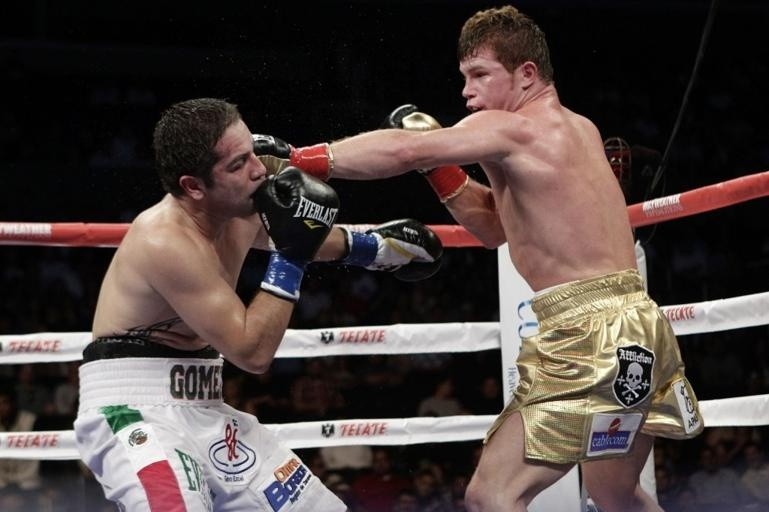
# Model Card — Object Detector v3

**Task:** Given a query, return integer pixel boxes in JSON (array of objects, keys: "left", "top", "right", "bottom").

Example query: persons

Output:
[
  {"left": 251, "top": 4, "right": 705, "bottom": 512},
  {"left": 71, "top": 96, "right": 445, "bottom": 512}
]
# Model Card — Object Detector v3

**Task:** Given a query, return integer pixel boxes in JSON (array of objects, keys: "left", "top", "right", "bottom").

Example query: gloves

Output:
[
  {"left": 389, "top": 104, "right": 468, "bottom": 202},
  {"left": 251, "top": 134, "right": 339, "bottom": 301},
  {"left": 340, "top": 218, "right": 443, "bottom": 282}
]
